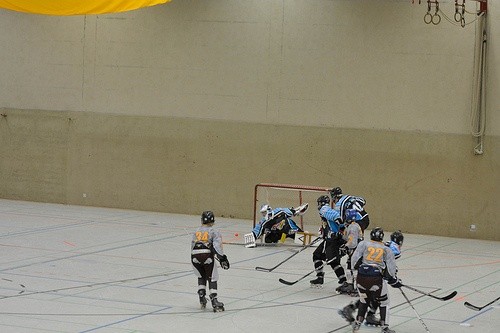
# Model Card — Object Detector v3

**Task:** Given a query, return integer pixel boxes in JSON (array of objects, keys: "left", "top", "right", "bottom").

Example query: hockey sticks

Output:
[
  {"left": 464, "top": 297, "right": 500, "bottom": 311},
  {"left": 214, "top": 256, "right": 228, "bottom": 269},
  {"left": 255, "top": 236, "right": 322, "bottom": 272},
  {"left": 400, "top": 284, "right": 458, "bottom": 301},
  {"left": 279, "top": 255, "right": 340, "bottom": 286},
  {"left": 399, "top": 287, "right": 430, "bottom": 333}
]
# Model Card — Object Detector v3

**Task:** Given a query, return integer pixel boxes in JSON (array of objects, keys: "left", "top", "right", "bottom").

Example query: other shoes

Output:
[
  {"left": 351, "top": 315, "right": 365, "bottom": 330},
  {"left": 379, "top": 326, "right": 396, "bottom": 333},
  {"left": 335, "top": 281, "right": 355, "bottom": 295}
]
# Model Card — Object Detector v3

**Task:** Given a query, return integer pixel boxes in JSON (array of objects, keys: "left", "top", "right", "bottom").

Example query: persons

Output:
[
  {"left": 190, "top": 210, "right": 230, "bottom": 312},
  {"left": 335, "top": 208, "right": 363, "bottom": 295},
  {"left": 337, "top": 227, "right": 406, "bottom": 333},
  {"left": 337, "top": 231, "right": 404, "bottom": 327},
  {"left": 244, "top": 203, "right": 310, "bottom": 245},
  {"left": 310, "top": 187, "right": 370, "bottom": 288}
]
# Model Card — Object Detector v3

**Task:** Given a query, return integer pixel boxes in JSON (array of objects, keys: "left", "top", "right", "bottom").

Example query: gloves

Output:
[
  {"left": 220, "top": 255, "right": 230, "bottom": 271},
  {"left": 337, "top": 244, "right": 348, "bottom": 258},
  {"left": 387, "top": 276, "right": 404, "bottom": 289}
]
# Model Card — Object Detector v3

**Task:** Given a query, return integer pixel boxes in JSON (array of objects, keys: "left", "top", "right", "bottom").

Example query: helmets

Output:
[
  {"left": 259, "top": 204, "right": 271, "bottom": 212},
  {"left": 201, "top": 211, "right": 214, "bottom": 224},
  {"left": 390, "top": 231, "right": 404, "bottom": 246},
  {"left": 370, "top": 227, "right": 385, "bottom": 242},
  {"left": 343, "top": 208, "right": 357, "bottom": 224},
  {"left": 328, "top": 186, "right": 342, "bottom": 202},
  {"left": 316, "top": 195, "right": 330, "bottom": 203}
]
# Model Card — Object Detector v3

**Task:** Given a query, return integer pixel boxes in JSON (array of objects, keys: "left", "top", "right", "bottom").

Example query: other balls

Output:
[{"left": 235, "top": 233, "right": 238, "bottom": 236}]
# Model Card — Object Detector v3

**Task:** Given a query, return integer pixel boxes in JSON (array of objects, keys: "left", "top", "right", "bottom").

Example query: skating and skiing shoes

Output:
[
  {"left": 363, "top": 312, "right": 383, "bottom": 327},
  {"left": 210, "top": 298, "right": 225, "bottom": 312},
  {"left": 199, "top": 292, "right": 208, "bottom": 311},
  {"left": 309, "top": 276, "right": 324, "bottom": 289},
  {"left": 337, "top": 305, "right": 355, "bottom": 325}
]
